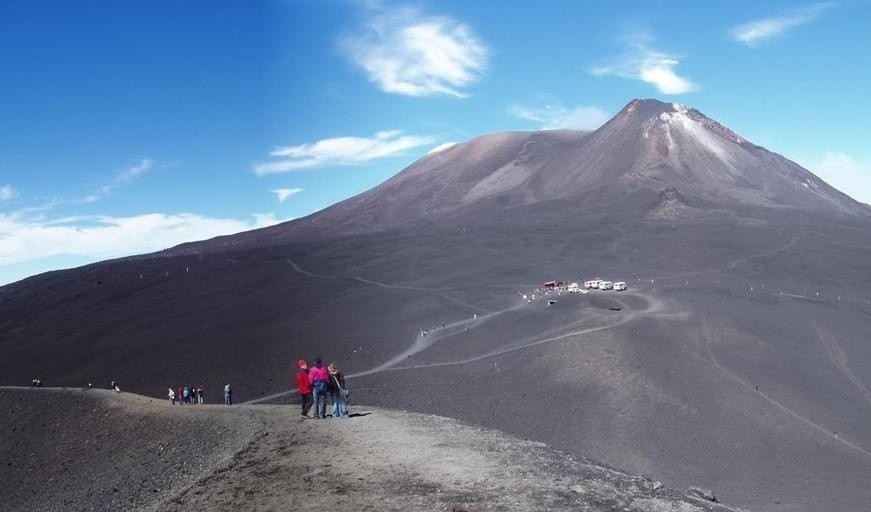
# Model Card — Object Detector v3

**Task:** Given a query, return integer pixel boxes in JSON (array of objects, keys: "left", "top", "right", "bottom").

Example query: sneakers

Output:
[{"left": 298, "top": 411, "right": 350, "bottom": 420}]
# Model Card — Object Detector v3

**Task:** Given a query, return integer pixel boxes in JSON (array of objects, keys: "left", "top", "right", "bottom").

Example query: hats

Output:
[{"left": 297, "top": 355, "right": 340, "bottom": 374}]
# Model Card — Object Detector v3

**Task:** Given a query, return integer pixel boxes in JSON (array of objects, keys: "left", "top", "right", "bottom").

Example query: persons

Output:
[
  {"left": 296, "top": 356, "right": 349, "bottom": 419},
  {"left": 168, "top": 384, "right": 204, "bottom": 406},
  {"left": 224, "top": 384, "right": 232, "bottom": 405}
]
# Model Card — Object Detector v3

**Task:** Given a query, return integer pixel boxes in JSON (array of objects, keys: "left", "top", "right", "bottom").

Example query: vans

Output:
[{"left": 544, "top": 279, "right": 628, "bottom": 295}]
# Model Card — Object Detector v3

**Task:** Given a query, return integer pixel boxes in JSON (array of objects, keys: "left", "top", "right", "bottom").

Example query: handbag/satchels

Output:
[
  {"left": 337, "top": 388, "right": 351, "bottom": 398},
  {"left": 311, "top": 379, "right": 329, "bottom": 394}
]
